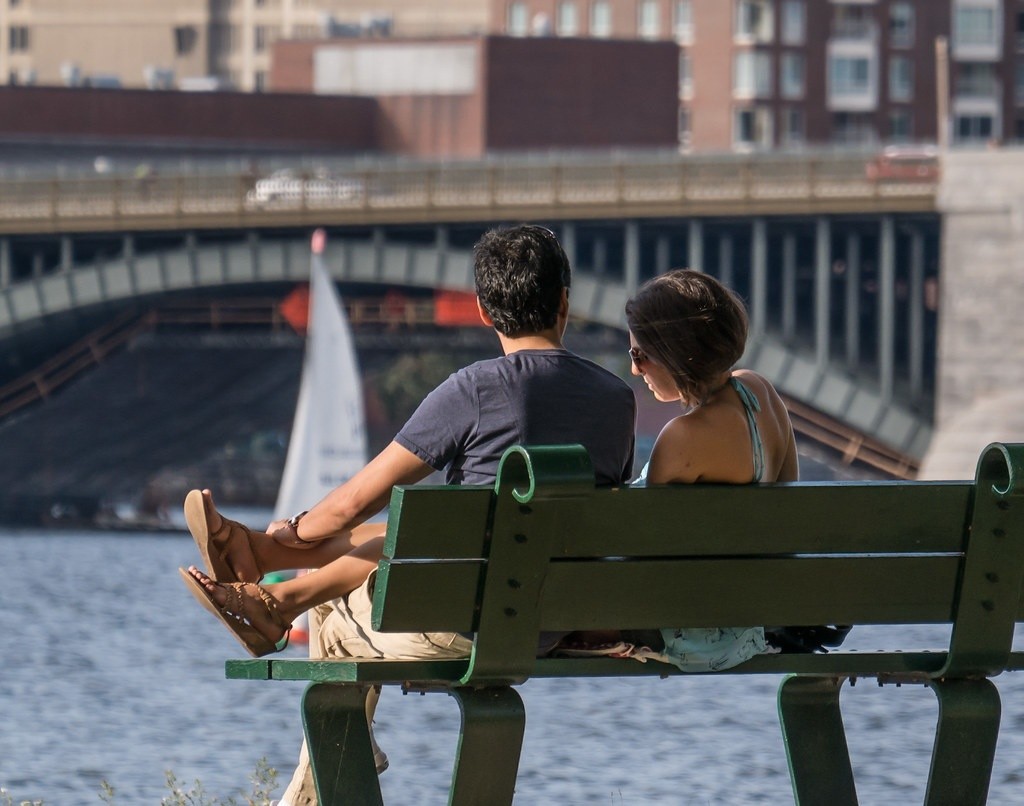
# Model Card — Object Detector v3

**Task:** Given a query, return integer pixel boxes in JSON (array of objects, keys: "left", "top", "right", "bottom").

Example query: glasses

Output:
[{"left": 628, "top": 347, "right": 649, "bottom": 368}]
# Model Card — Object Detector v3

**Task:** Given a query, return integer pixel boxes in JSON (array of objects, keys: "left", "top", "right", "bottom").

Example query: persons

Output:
[
  {"left": 267, "top": 223, "right": 637, "bottom": 776},
  {"left": 178, "top": 268, "right": 800, "bottom": 671}
]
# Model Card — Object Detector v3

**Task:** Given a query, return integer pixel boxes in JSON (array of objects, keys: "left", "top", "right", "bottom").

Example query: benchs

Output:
[{"left": 226, "top": 440, "right": 1024, "bottom": 806}]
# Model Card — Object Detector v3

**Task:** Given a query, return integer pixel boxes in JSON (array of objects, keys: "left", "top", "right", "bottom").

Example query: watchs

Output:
[{"left": 288, "top": 510, "right": 317, "bottom": 544}]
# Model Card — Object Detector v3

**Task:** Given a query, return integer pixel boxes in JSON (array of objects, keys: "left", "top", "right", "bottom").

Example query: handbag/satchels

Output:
[{"left": 770, "top": 623, "right": 852, "bottom": 653}]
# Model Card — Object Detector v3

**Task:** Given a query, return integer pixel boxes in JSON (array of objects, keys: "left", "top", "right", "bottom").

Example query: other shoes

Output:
[{"left": 371, "top": 735, "right": 388, "bottom": 775}]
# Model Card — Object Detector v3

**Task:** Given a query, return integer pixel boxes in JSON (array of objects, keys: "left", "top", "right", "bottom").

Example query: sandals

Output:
[
  {"left": 178, "top": 567, "right": 294, "bottom": 658},
  {"left": 184, "top": 489, "right": 265, "bottom": 586}
]
